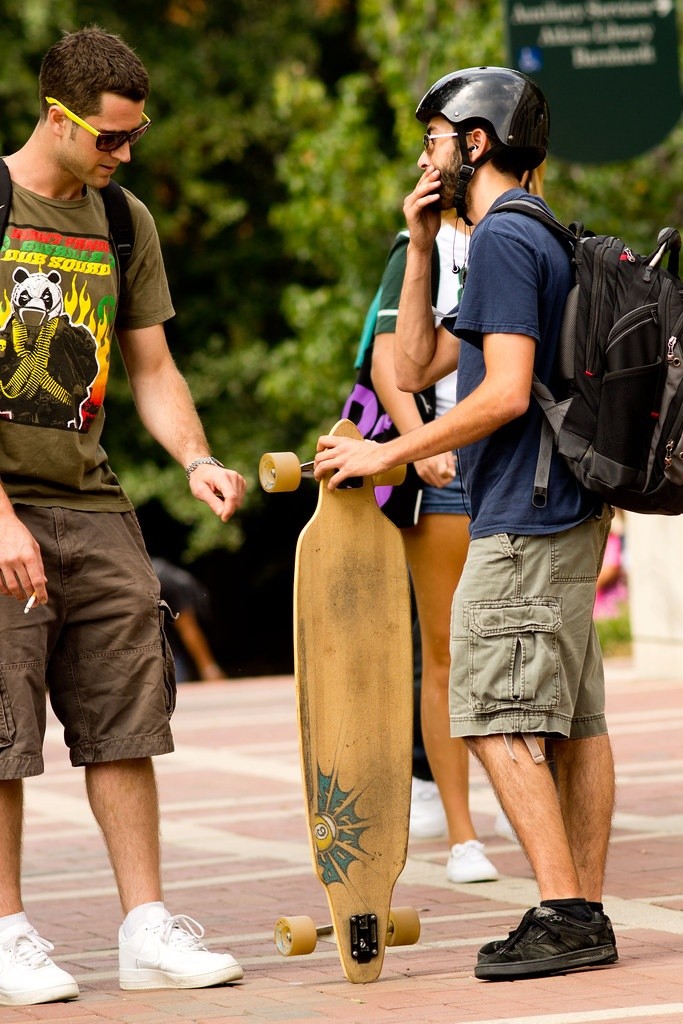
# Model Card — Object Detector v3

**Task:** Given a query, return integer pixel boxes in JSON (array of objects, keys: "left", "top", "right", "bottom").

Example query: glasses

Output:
[
  {"left": 45, "top": 97, "right": 152, "bottom": 152},
  {"left": 423, "top": 131, "right": 496, "bottom": 153}
]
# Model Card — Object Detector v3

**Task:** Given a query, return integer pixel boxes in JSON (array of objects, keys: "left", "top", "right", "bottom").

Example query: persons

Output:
[
  {"left": 0, "top": 26, "right": 246, "bottom": 1007},
  {"left": 594, "top": 509, "right": 625, "bottom": 619},
  {"left": 314, "top": 67, "right": 619, "bottom": 979},
  {"left": 151, "top": 557, "right": 223, "bottom": 681},
  {"left": 370, "top": 160, "right": 543, "bottom": 882},
  {"left": 352, "top": 285, "right": 522, "bottom": 841}
]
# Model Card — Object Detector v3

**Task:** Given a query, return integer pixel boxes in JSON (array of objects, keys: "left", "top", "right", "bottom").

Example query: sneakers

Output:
[
  {"left": 0, "top": 922, "right": 80, "bottom": 1006},
  {"left": 118, "top": 907, "right": 243, "bottom": 990},
  {"left": 474, "top": 906, "right": 620, "bottom": 980},
  {"left": 446, "top": 839, "right": 497, "bottom": 883},
  {"left": 493, "top": 809, "right": 520, "bottom": 842}
]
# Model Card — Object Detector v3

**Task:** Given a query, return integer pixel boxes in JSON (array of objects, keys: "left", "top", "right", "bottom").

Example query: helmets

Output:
[{"left": 414, "top": 66, "right": 551, "bottom": 153}]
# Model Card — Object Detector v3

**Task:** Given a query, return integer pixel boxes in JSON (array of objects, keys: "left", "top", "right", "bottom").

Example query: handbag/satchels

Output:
[{"left": 339, "top": 345, "right": 435, "bottom": 527}]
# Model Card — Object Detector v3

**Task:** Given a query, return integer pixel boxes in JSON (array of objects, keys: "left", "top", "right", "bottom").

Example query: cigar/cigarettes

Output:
[{"left": 24, "top": 592, "right": 36, "bottom": 614}]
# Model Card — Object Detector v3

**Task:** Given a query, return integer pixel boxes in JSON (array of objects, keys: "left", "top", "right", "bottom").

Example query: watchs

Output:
[{"left": 186, "top": 456, "right": 225, "bottom": 480}]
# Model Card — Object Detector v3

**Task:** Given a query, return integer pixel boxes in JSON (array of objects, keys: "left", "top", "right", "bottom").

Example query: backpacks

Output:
[{"left": 488, "top": 200, "right": 683, "bottom": 516}]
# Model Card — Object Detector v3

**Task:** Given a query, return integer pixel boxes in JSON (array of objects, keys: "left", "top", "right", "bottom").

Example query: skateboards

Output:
[{"left": 258, "top": 416, "right": 422, "bottom": 985}]
